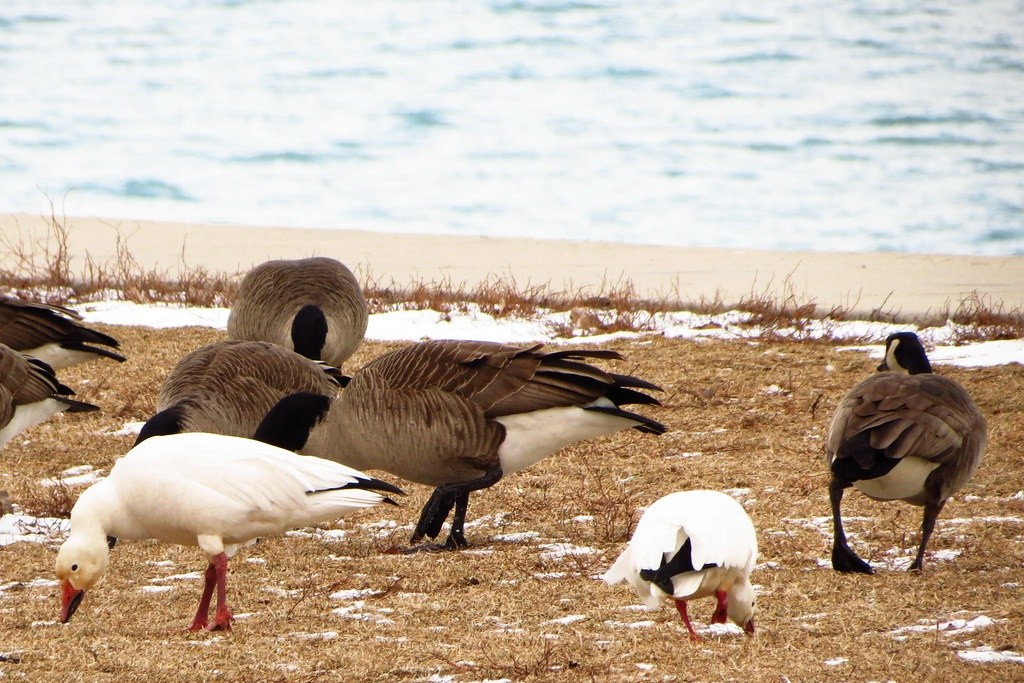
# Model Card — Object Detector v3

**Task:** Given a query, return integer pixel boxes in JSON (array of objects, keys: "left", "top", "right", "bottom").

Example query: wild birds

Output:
[
  {"left": 602, "top": 487, "right": 760, "bottom": 644},
  {"left": 0, "top": 294, "right": 126, "bottom": 453},
  {"left": 821, "top": 331, "right": 986, "bottom": 575},
  {"left": 51, "top": 258, "right": 669, "bottom": 636}
]
